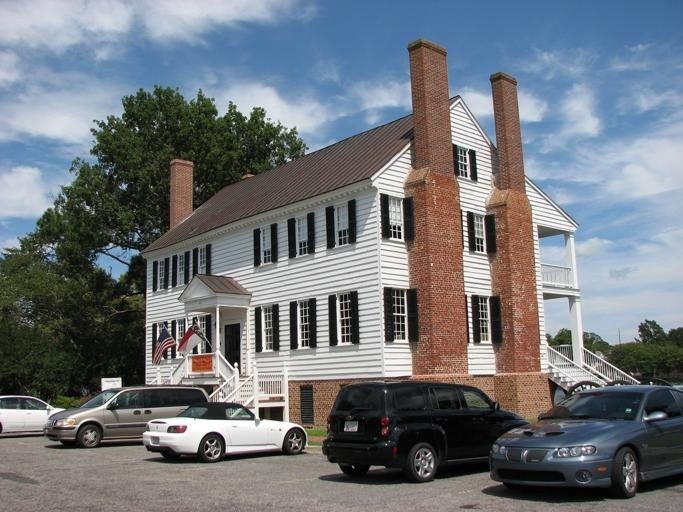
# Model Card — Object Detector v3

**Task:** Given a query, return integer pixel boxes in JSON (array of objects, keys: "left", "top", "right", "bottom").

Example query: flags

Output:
[
  {"left": 151, "top": 329, "right": 176, "bottom": 365},
  {"left": 177, "top": 326, "right": 206, "bottom": 356}
]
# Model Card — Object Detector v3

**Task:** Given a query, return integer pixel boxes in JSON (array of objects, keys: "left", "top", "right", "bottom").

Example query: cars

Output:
[
  {"left": 487, "top": 384, "right": 682, "bottom": 499},
  {"left": 142, "top": 402, "right": 307, "bottom": 462},
  {"left": 0, "top": 395, "right": 66, "bottom": 438}
]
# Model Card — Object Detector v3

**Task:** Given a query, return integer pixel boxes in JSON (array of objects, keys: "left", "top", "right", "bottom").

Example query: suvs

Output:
[{"left": 322, "top": 380, "right": 532, "bottom": 483}]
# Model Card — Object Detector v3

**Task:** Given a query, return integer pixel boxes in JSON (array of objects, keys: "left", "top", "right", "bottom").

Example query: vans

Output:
[{"left": 43, "top": 385, "right": 210, "bottom": 448}]
